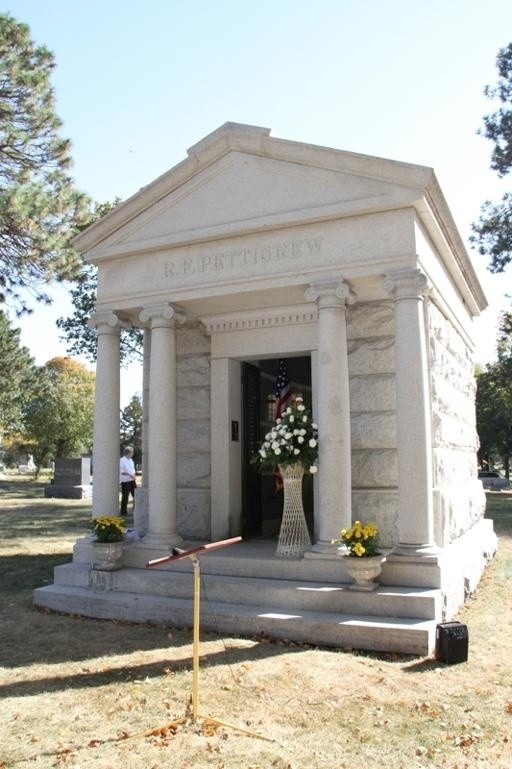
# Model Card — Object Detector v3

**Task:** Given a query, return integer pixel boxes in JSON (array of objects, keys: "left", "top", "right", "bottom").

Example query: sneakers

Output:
[{"left": 121, "top": 511, "right": 128, "bottom": 516}]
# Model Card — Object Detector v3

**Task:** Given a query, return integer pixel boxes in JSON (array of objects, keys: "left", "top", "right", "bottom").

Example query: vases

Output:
[
  {"left": 343, "top": 554, "right": 388, "bottom": 592},
  {"left": 90, "top": 542, "right": 124, "bottom": 571}
]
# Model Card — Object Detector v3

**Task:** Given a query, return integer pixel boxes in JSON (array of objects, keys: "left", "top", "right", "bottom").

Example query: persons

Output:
[{"left": 119, "top": 445, "right": 138, "bottom": 517}]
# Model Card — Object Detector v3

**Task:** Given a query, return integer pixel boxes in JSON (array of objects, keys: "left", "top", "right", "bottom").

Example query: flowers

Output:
[
  {"left": 330, "top": 520, "right": 380, "bottom": 558},
  {"left": 89, "top": 515, "right": 129, "bottom": 541},
  {"left": 248, "top": 396, "right": 321, "bottom": 476}
]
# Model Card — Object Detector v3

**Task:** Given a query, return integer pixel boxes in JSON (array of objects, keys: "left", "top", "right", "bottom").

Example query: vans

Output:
[{"left": 478, "top": 471, "right": 509, "bottom": 489}]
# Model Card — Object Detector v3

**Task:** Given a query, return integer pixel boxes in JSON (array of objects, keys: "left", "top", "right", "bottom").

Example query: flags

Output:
[{"left": 272, "top": 358, "right": 295, "bottom": 492}]
